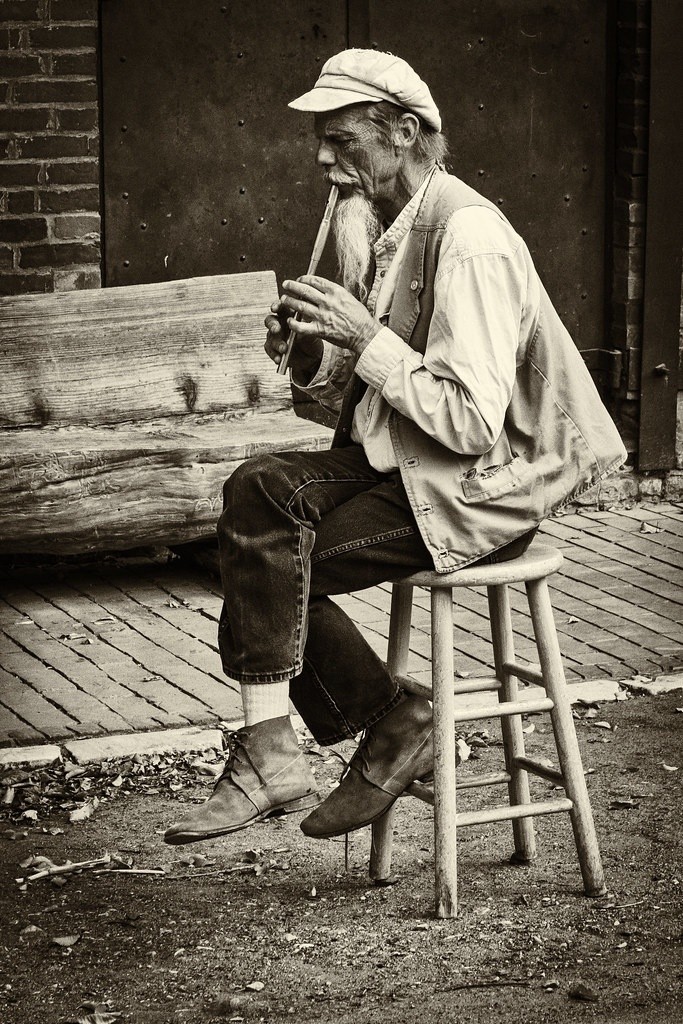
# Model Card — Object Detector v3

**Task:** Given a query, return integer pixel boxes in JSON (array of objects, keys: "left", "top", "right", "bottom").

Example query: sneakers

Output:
[{"left": 163, "top": 715, "right": 321, "bottom": 844}]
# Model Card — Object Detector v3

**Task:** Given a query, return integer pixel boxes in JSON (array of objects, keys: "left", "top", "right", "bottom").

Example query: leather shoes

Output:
[
  {"left": 251, "top": 773, "right": 257, "bottom": 775},
  {"left": 301, "top": 692, "right": 461, "bottom": 838}
]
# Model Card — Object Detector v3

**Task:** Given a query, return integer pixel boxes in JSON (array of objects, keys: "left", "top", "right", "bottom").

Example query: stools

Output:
[{"left": 367, "top": 542, "right": 608, "bottom": 918}]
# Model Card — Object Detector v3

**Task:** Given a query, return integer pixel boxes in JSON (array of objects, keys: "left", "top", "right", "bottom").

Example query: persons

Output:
[{"left": 163, "top": 47, "right": 629, "bottom": 845}]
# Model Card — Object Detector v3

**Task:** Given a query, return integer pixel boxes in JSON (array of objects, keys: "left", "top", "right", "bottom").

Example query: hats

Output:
[{"left": 288, "top": 48, "right": 441, "bottom": 133}]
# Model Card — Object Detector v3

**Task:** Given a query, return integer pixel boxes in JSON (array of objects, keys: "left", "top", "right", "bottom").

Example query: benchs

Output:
[{"left": 0, "top": 271, "right": 335, "bottom": 569}]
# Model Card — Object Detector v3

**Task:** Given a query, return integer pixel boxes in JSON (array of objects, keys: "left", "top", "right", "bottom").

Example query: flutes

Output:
[{"left": 276, "top": 184, "right": 338, "bottom": 377}]
